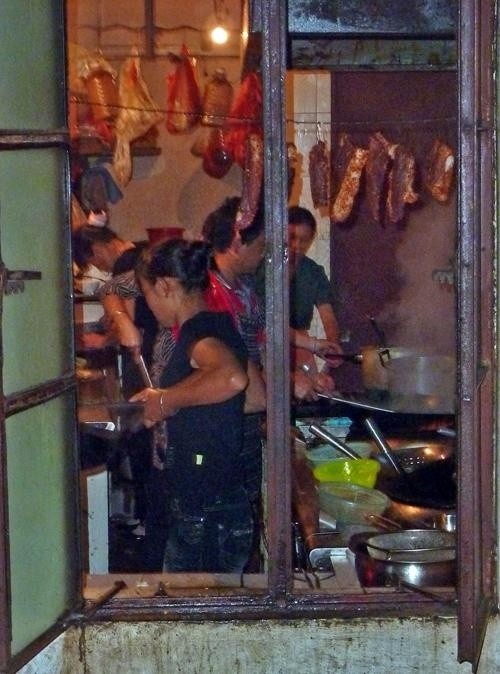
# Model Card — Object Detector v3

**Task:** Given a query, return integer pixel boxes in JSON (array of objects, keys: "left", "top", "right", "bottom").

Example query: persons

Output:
[
  {"left": 285, "top": 204, "right": 341, "bottom": 374},
  {"left": 172, "top": 197, "right": 342, "bottom": 413},
  {"left": 73, "top": 221, "right": 158, "bottom": 536},
  {"left": 128, "top": 237, "right": 272, "bottom": 572},
  {"left": 100, "top": 264, "right": 178, "bottom": 575}
]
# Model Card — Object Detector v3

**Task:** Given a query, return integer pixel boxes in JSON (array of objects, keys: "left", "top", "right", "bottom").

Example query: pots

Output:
[
  {"left": 301, "top": 362, "right": 457, "bottom": 435},
  {"left": 76, "top": 407, "right": 151, "bottom": 469},
  {"left": 308, "top": 423, "right": 459, "bottom": 510},
  {"left": 324, "top": 345, "right": 417, "bottom": 389},
  {"left": 375, "top": 348, "right": 458, "bottom": 400}
]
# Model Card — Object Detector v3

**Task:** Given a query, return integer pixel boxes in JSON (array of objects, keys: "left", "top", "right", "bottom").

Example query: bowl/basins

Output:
[
  {"left": 313, "top": 459, "right": 382, "bottom": 499},
  {"left": 315, "top": 482, "right": 390, "bottom": 525},
  {"left": 305, "top": 441, "right": 372, "bottom": 465},
  {"left": 76, "top": 279, "right": 100, "bottom": 295}
]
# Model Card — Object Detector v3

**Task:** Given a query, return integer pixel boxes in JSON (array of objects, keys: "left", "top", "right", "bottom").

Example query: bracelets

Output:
[
  {"left": 161, "top": 390, "right": 181, "bottom": 417},
  {"left": 108, "top": 308, "right": 128, "bottom": 316},
  {"left": 310, "top": 337, "right": 317, "bottom": 352}
]
